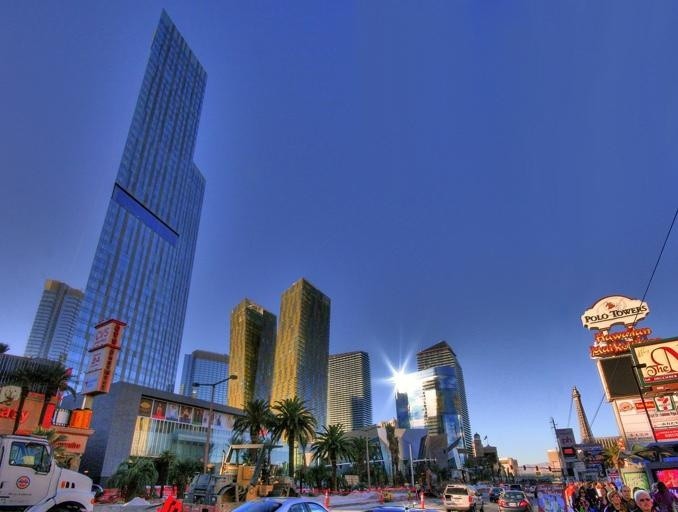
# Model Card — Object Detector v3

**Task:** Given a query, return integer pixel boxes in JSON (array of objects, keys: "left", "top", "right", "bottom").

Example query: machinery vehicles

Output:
[{"left": 183, "top": 443, "right": 301, "bottom": 512}]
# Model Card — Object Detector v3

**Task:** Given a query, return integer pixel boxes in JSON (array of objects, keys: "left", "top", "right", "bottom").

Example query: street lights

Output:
[
  {"left": 366, "top": 423, "right": 383, "bottom": 488},
  {"left": 192, "top": 375, "right": 237, "bottom": 475}
]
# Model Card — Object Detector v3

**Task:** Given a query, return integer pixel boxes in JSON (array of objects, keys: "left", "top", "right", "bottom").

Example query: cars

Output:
[
  {"left": 442, "top": 480, "right": 535, "bottom": 511},
  {"left": 232, "top": 497, "right": 332, "bottom": 512}
]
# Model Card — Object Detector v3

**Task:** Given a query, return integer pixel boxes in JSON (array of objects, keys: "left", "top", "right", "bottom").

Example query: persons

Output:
[{"left": 563, "top": 474, "right": 677, "bottom": 512}]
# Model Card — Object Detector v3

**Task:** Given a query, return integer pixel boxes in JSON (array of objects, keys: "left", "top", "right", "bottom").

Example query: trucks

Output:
[{"left": 0, "top": 435, "right": 102, "bottom": 512}]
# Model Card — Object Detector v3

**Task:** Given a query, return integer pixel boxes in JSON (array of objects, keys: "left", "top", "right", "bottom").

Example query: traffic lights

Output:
[{"left": 424, "top": 458, "right": 437, "bottom": 463}]
